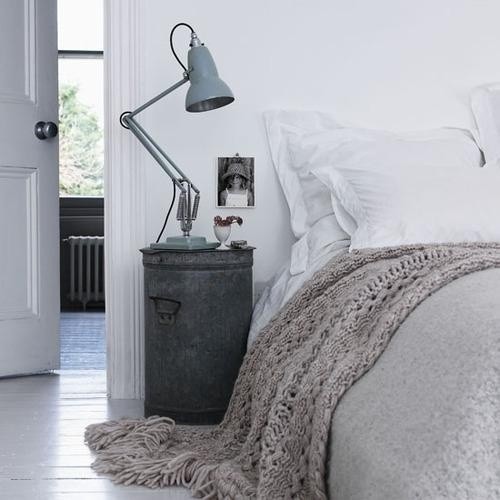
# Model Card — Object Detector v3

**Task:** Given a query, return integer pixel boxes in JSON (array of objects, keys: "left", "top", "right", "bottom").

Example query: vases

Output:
[{"left": 213, "top": 226, "right": 231, "bottom": 250}]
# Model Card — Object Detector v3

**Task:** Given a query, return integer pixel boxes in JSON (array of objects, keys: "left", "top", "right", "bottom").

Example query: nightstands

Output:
[{"left": 140, "top": 246, "right": 257, "bottom": 426}]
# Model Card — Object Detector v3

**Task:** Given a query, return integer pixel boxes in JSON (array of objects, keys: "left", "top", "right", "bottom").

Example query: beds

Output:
[{"left": 248, "top": 244, "right": 500, "bottom": 500}]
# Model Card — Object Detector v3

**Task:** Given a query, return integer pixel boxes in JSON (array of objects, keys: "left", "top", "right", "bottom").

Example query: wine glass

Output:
[{"left": 213, "top": 225, "right": 231, "bottom": 250}]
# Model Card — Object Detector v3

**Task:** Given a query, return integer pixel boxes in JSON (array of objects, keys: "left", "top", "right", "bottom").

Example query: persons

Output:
[{"left": 219, "top": 163, "right": 255, "bottom": 206}]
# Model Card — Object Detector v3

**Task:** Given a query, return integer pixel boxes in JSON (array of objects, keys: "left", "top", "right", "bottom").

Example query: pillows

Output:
[{"left": 263, "top": 82, "right": 500, "bottom": 253}]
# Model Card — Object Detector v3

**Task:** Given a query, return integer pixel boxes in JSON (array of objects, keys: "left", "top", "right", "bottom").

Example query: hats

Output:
[{"left": 222, "top": 163, "right": 248, "bottom": 182}]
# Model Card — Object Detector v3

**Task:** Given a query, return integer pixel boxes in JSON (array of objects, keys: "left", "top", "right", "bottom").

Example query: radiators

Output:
[{"left": 61, "top": 235, "right": 105, "bottom": 311}]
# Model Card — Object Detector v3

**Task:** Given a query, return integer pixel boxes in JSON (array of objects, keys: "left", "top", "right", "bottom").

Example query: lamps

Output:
[{"left": 119, "top": 23, "right": 235, "bottom": 252}]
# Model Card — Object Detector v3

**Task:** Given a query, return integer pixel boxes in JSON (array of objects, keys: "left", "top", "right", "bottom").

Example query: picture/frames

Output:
[{"left": 216, "top": 156, "right": 256, "bottom": 209}]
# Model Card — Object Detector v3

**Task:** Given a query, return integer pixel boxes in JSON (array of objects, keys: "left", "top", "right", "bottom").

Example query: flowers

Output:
[{"left": 214, "top": 215, "right": 243, "bottom": 227}]
